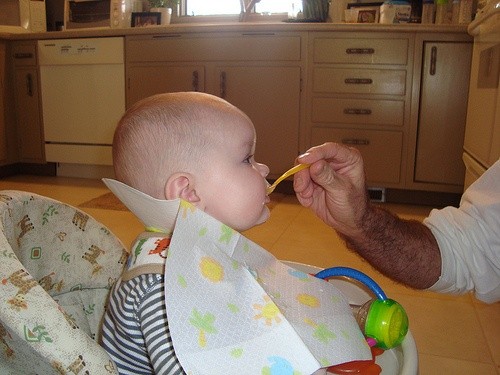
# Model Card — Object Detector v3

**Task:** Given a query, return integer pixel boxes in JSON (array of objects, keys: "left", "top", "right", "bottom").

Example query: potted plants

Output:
[{"left": 147, "top": 0, "right": 182, "bottom": 25}]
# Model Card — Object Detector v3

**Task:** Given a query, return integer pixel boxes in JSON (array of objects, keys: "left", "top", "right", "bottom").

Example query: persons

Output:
[
  {"left": 293, "top": 142, "right": 500, "bottom": 306},
  {"left": 101, "top": 92, "right": 274, "bottom": 375}
]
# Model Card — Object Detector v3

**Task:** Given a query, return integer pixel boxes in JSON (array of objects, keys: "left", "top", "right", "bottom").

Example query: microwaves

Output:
[
  {"left": 0, "top": 0, "right": 47, "bottom": 33},
  {"left": 63, "top": 0, "right": 142, "bottom": 31}
]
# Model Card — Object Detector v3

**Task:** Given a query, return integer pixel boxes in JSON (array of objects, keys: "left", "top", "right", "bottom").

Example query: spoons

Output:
[{"left": 266, "top": 163, "right": 310, "bottom": 194}]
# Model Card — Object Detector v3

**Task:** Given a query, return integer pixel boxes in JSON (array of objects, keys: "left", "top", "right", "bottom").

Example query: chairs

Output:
[{"left": 0, "top": 188, "right": 418, "bottom": 375}]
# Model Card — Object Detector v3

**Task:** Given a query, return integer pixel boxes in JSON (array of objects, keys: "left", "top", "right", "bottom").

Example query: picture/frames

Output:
[
  {"left": 346, "top": 3, "right": 383, "bottom": 23},
  {"left": 130, "top": 12, "right": 161, "bottom": 28}
]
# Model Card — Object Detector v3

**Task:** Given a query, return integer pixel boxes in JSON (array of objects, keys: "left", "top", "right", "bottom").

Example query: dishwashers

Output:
[{"left": 37, "top": 36, "right": 126, "bottom": 179}]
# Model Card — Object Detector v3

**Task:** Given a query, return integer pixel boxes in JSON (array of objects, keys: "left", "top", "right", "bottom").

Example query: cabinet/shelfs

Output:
[
  {"left": 462, "top": 3, "right": 500, "bottom": 199},
  {"left": 124, "top": 29, "right": 473, "bottom": 209},
  {"left": 0, "top": 38, "right": 56, "bottom": 177}
]
